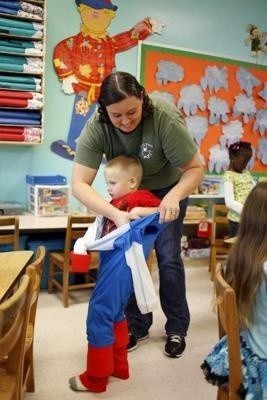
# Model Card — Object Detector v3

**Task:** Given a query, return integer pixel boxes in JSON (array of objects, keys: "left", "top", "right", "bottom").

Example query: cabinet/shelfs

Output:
[
  {"left": 0, "top": 0, "right": 47, "bottom": 146},
  {"left": 180, "top": 193, "right": 224, "bottom": 263}
]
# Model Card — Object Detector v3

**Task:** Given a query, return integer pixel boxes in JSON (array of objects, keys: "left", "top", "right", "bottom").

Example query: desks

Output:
[{"left": 224, "top": 236, "right": 236, "bottom": 245}]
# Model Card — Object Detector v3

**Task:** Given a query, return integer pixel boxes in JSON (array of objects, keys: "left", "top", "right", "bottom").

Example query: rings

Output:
[{"left": 171, "top": 212, "right": 175, "bottom": 215}]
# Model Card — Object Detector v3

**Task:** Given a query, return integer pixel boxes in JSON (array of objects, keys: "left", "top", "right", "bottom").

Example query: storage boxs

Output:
[{"left": 27, "top": 184, "right": 72, "bottom": 216}]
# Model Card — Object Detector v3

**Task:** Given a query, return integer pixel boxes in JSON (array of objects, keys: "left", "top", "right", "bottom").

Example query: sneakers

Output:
[
  {"left": 164, "top": 334, "right": 186, "bottom": 357},
  {"left": 127, "top": 329, "right": 149, "bottom": 351}
]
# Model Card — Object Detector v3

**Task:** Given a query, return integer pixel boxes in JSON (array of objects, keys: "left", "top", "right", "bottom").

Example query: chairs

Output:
[
  {"left": 208, "top": 203, "right": 234, "bottom": 281},
  {"left": 0, "top": 207, "right": 102, "bottom": 400},
  {"left": 213, "top": 262, "right": 247, "bottom": 400}
]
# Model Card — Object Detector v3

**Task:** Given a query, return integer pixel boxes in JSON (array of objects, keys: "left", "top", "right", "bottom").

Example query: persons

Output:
[
  {"left": 221, "top": 141, "right": 257, "bottom": 237},
  {"left": 70, "top": 70, "right": 205, "bottom": 358},
  {"left": 68, "top": 154, "right": 169, "bottom": 394},
  {"left": 200, "top": 181, "right": 267, "bottom": 400}
]
